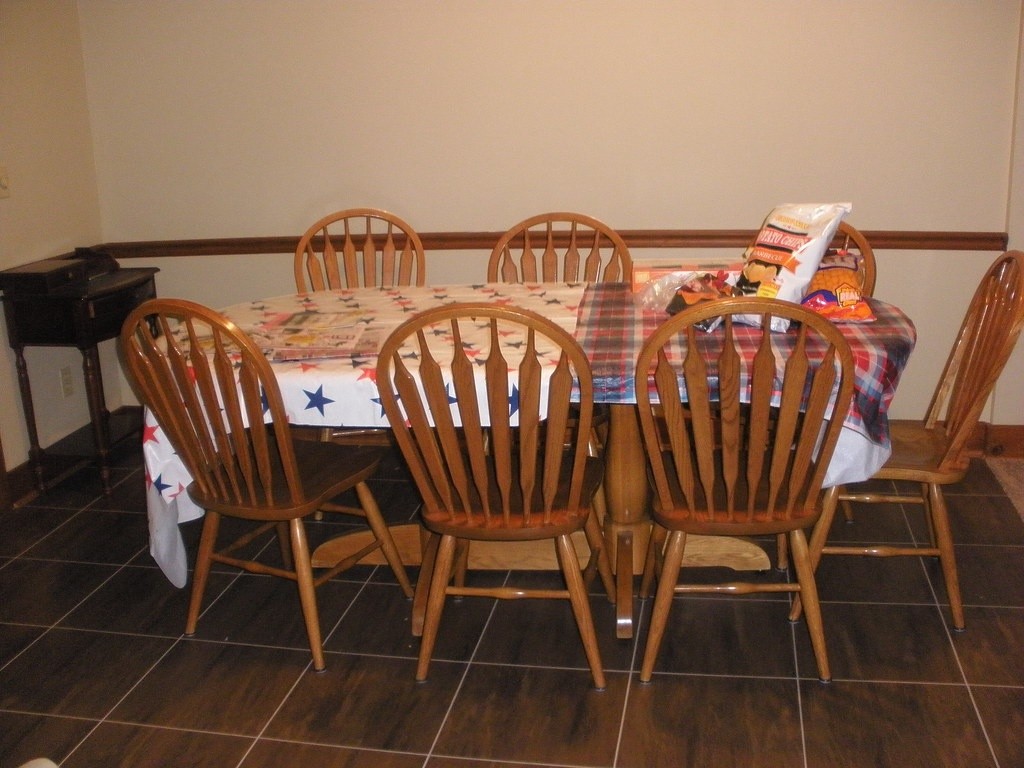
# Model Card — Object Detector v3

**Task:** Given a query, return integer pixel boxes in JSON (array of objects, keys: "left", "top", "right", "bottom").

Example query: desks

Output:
[{"left": 1, "top": 243, "right": 161, "bottom": 497}]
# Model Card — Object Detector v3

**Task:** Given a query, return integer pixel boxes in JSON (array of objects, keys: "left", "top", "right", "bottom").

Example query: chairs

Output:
[
  {"left": 654, "top": 219, "right": 877, "bottom": 525},
  {"left": 375, "top": 301, "right": 618, "bottom": 692},
  {"left": 294, "top": 207, "right": 426, "bottom": 524},
  {"left": 117, "top": 298, "right": 415, "bottom": 671},
  {"left": 482, "top": 212, "right": 632, "bottom": 530},
  {"left": 787, "top": 250, "right": 1024, "bottom": 633},
  {"left": 635, "top": 297, "right": 855, "bottom": 684}
]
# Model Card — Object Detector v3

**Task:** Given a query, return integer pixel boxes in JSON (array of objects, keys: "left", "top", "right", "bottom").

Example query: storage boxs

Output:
[{"left": 632, "top": 258, "right": 744, "bottom": 291}]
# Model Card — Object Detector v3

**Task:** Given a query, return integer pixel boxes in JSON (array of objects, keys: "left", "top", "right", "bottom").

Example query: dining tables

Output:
[{"left": 143, "top": 281, "right": 918, "bottom": 574}]
